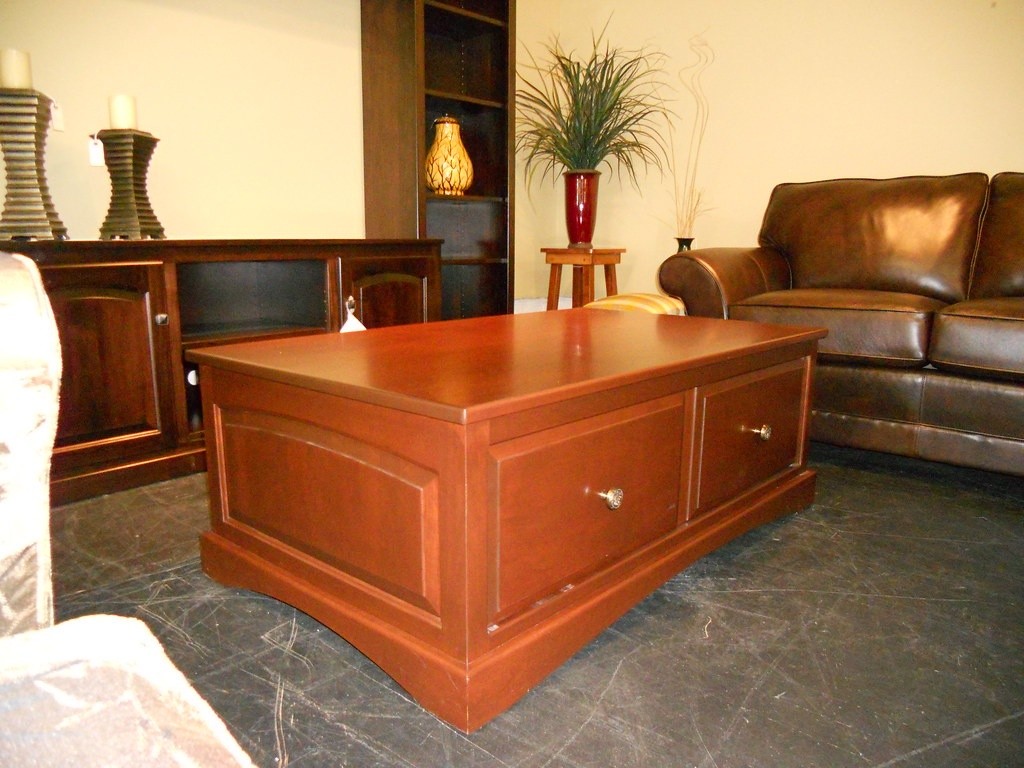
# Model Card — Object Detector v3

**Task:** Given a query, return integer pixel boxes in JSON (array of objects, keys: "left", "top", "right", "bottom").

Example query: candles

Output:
[
  {"left": 0, "top": 48, "right": 34, "bottom": 90},
  {"left": 108, "top": 93, "right": 139, "bottom": 130}
]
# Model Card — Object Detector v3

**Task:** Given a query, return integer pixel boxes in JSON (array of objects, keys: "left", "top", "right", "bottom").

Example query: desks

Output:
[{"left": 0, "top": 613, "right": 260, "bottom": 768}]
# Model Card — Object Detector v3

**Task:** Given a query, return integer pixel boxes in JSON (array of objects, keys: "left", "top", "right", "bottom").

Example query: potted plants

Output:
[{"left": 515, "top": 28, "right": 682, "bottom": 248}]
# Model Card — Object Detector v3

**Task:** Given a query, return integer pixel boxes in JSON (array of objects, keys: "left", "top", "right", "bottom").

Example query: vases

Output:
[
  {"left": 674, "top": 238, "right": 695, "bottom": 252},
  {"left": 425, "top": 117, "right": 476, "bottom": 195}
]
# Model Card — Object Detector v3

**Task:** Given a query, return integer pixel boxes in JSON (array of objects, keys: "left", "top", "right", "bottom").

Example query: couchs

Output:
[{"left": 658, "top": 171, "right": 1024, "bottom": 478}]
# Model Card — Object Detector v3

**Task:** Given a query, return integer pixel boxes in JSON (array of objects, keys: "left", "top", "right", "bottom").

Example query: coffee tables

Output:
[{"left": 180, "top": 305, "right": 829, "bottom": 736}]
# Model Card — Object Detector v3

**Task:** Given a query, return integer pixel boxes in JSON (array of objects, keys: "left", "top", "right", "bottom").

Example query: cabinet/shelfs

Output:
[
  {"left": 359, "top": 0, "right": 517, "bottom": 320},
  {"left": 0, "top": 238, "right": 445, "bottom": 509}
]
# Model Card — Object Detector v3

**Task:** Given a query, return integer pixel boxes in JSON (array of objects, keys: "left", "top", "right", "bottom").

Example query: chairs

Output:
[{"left": 0, "top": 254, "right": 65, "bottom": 637}]
